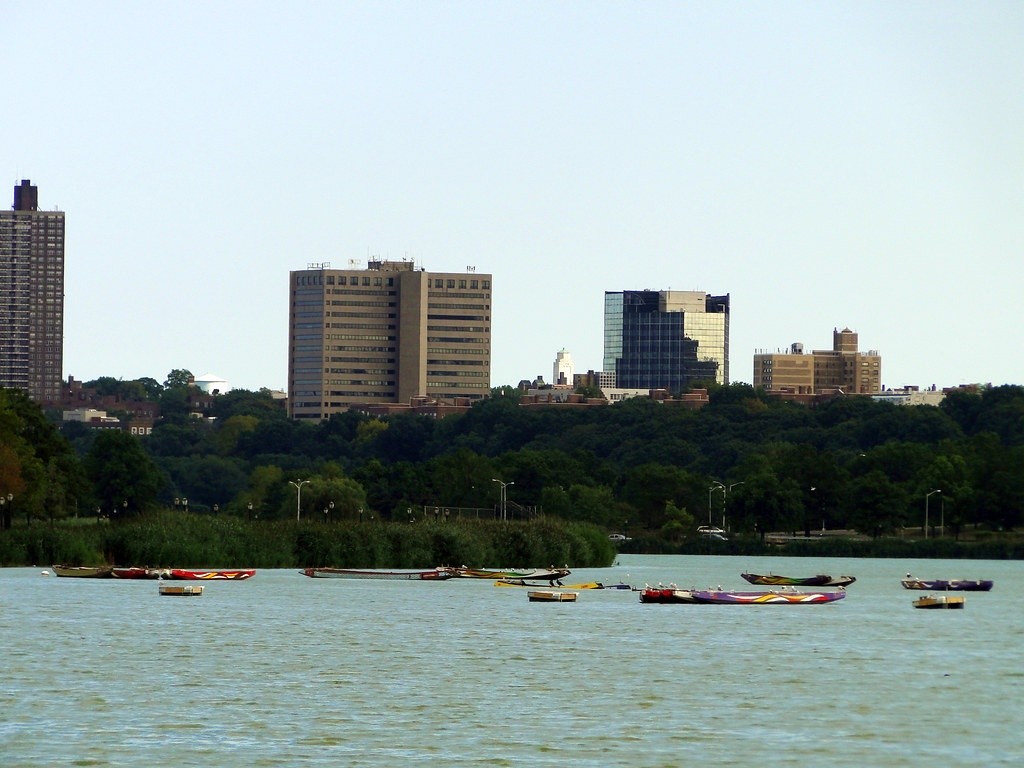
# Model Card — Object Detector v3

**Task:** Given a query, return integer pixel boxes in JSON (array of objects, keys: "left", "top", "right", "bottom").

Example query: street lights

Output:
[
  {"left": 925, "top": 490, "right": 942, "bottom": 540},
  {"left": 728, "top": 482, "right": 744, "bottom": 531},
  {"left": 709, "top": 486, "right": 722, "bottom": 524},
  {"left": 492, "top": 479, "right": 515, "bottom": 522},
  {"left": 288, "top": 480, "right": 311, "bottom": 522},
  {"left": 713, "top": 481, "right": 725, "bottom": 527}
]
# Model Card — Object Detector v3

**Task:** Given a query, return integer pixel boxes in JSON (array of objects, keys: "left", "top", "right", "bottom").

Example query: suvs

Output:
[{"left": 697, "top": 526, "right": 726, "bottom": 534}]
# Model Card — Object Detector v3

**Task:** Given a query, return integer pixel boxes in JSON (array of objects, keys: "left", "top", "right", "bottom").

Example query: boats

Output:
[
  {"left": 902, "top": 579, "right": 993, "bottom": 592},
  {"left": 436, "top": 566, "right": 571, "bottom": 580},
  {"left": 739, "top": 571, "right": 855, "bottom": 587},
  {"left": 53, "top": 564, "right": 114, "bottom": 577},
  {"left": 642, "top": 587, "right": 846, "bottom": 605},
  {"left": 79, "top": 567, "right": 256, "bottom": 581},
  {"left": 494, "top": 580, "right": 631, "bottom": 590},
  {"left": 298, "top": 567, "right": 459, "bottom": 580},
  {"left": 528, "top": 592, "right": 577, "bottom": 601},
  {"left": 158, "top": 586, "right": 204, "bottom": 597},
  {"left": 912, "top": 596, "right": 964, "bottom": 609}
]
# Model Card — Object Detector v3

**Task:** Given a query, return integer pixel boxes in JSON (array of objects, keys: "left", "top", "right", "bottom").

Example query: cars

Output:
[
  {"left": 705, "top": 534, "right": 728, "bottom": 540},
  {"left": 608, "top": 534, "right": 632, "bottom": 542}
]
